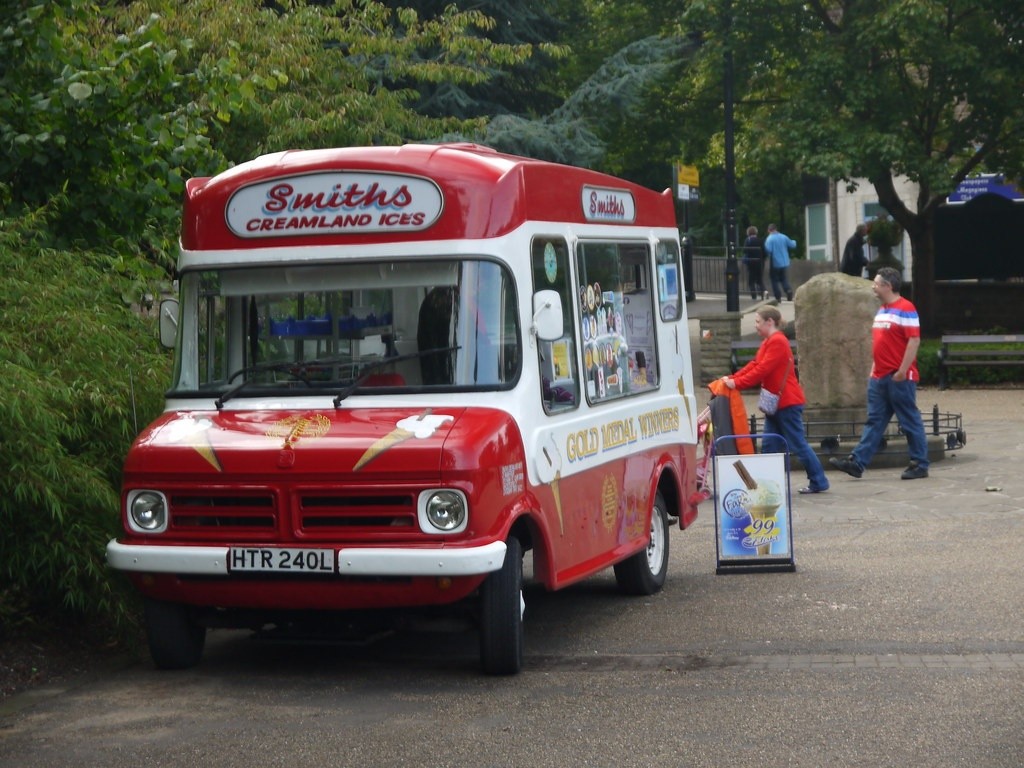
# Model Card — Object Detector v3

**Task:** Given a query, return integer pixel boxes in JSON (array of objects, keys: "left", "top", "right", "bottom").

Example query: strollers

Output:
[{"left": 696, "top": 379, "right": 722, "bottom": 501}]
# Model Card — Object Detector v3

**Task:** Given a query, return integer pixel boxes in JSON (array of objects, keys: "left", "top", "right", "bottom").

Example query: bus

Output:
[{"left": 102, "top": 141, "right": 701, "bottom": 673}]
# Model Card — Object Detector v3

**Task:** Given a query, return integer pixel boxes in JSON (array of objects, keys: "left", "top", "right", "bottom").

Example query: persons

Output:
[
  {"left": 721, "top": 304, "right": 830, "bottom": 494},
  {"left": 418, "top": 257, "right": 489, "bottom": 382},
  {"left": 765, "top": 223, "right": 797, "bottom": 304},
  {"left": 841, "top": 224, "right": 869, "bottom": 277},
  {"left": 743, "top": 226, "right": 769, "bottom": 301},
  {"left": 828, "top": 267, "right": 929, "bottom": 480}
]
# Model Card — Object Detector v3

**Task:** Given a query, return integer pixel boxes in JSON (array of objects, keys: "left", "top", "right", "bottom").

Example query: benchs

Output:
[
  {"left": 729, "top": 339, "right": 798, "bottom": 389},
  {"left": 935, "top": 335, "right": 1024, "bottom": 391}
]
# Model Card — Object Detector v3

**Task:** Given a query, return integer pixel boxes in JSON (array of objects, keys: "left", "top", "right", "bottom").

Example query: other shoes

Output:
[
  {"left": 798, "top": 486, "right": 817, "bottom": 493},
  {"left": 786, "top": 290, "right": 793, "bottom": 301},
  {"left": 776, "top": 298, "right": 781, "bottom": 303},
  {"left": 763, "top": 290, "right": 769, "bottom": 300}
]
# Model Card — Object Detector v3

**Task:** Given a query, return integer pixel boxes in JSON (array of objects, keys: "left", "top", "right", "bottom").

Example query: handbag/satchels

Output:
[
  {"left": 757, "top": 387, "right": 780, "bottom": 416},
  {"left": 742, "top": 253, "right": 751, "bottom": 265}
]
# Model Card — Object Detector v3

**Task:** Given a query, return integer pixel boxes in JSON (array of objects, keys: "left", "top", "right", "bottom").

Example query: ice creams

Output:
[
  {"left": 543, "top": 434, "right": 563, "bottom": 538},
  {"left": 354, "top": 407, "right": 454, "bottom": 470},
  {"left": 732, "top": 459, "right": 782, "bottom": 556},
  {"left": 669, "top": 326, "right": 694, "bottom": 439},
  {"left": 164, "top": 416, "right": 223, "bottom": 472}
]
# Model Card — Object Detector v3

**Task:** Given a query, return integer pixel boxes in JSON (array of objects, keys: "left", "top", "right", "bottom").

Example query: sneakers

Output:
[
  {"left": 901, "top": 464, "right": 928, "bottom": 479},
  {"left": 829, "top": 455, "right": 862, "bottom": 478}
]
